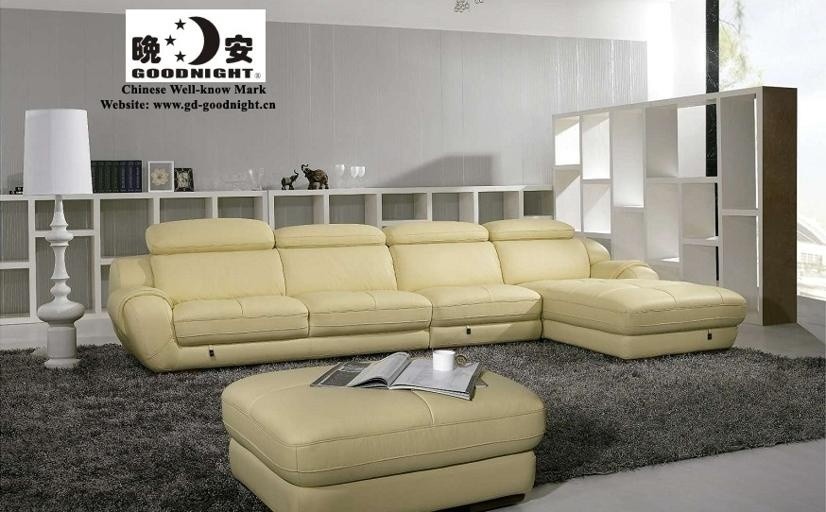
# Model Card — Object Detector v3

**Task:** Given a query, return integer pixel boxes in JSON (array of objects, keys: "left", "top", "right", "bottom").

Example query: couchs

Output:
[{"left": 106, "top": 213, "right": 748, "bottom": 371}]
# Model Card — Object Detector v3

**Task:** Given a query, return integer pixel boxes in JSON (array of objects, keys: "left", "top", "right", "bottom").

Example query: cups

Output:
[
  {"left": 431, "top": 349, "right": 455, "bottom": 370},
  {"left": 249, "top": 168, "right": 264, "bottom": 192}
]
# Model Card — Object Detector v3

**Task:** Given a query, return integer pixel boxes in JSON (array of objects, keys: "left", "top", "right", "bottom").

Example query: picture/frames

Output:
[{"left": 146, "top": 160, "right": 195, "bottom": 193}]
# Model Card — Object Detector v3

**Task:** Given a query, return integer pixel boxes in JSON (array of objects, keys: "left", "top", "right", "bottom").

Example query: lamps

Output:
[{"left": 20, "top": 107, "right": 97, "bottom": 369}]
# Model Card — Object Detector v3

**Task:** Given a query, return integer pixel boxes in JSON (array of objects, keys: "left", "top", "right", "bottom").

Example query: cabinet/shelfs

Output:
[
  {"left": 0, "top": 184, "right": 559, "bottom": 353},
  {"left": 552, "top": 84, "right": 798, "bottom": 327}
]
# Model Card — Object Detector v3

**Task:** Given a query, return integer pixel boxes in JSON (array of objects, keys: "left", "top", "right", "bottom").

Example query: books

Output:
[
  {"left": 311, "top": 351, "right": 482, "bottom": 401},
  {"left": 92, "top": 160, "right": 143, "bottom": 192}
]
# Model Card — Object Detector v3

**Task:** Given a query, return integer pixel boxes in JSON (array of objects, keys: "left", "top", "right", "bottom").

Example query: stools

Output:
[{"left": 219, "top": 360, "right": 547, "bottom": 511}]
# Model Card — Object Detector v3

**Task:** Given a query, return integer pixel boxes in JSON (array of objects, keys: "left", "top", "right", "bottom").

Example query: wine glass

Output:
[{"left": 335, "top": 163, "right": 366, "bottom": 187}]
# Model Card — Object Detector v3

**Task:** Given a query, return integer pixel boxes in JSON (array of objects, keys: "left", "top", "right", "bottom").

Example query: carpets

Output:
[{"left": 0, "top": 343, "right": 826, "bottom": 511}]
